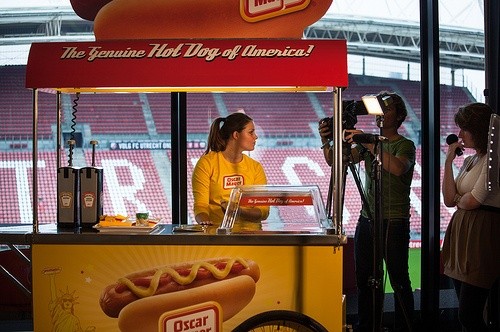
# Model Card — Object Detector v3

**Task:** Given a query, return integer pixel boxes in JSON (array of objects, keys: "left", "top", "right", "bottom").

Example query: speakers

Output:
[
  {"left": 57, "top": 167, "right": 80, "bottom": 233},
  {"left": 79, "top": 167, "right": 103, "bottom": 233}
]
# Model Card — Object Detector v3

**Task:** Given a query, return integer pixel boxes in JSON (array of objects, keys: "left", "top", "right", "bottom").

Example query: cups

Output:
[{"left": 136, "top": 213, "right": 149, "bottom": 227}]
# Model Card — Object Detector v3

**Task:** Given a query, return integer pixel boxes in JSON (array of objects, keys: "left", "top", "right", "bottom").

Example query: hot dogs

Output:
[{"left": 98, "top": 256, "right": 260, "bottom": 332}]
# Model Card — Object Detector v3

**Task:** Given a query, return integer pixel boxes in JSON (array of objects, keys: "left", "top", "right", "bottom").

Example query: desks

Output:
[{"left": 0, "top": 225, "right": 45, "bottom": 301}]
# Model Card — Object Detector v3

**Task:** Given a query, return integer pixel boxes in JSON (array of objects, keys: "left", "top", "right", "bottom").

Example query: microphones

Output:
[
  {"left": 353, "top": 134, "right": 388, "bottom": 143},
  {"left": 447, "top": 134, "right": 463, "bottom": 157}
]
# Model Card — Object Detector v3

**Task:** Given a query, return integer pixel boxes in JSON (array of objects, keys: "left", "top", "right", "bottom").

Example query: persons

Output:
[
  {"left": 193, "top": 113, "right": 270, "bottom": 226},
  {"left": 320, "top": 91, "right": 415, "bottom": 332},
  {"left": 442, "top": 102, "right": 500, "bottom": 332}
]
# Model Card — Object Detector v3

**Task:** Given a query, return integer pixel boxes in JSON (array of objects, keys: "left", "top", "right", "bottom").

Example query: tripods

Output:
[{"left": 321, "top": 129, "right": 374, "bottom": 228}]
